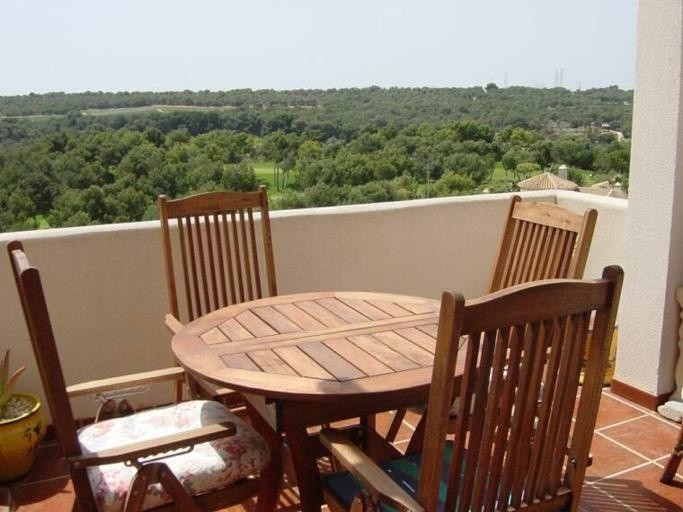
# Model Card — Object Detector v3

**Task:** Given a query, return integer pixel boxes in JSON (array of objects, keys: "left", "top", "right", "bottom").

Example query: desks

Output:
[{"left": 165, "top": 291, "right": 510, "bottom": 505}]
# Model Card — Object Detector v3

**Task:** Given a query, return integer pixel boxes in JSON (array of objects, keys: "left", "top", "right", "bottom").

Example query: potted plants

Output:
[{"left": 0, "top": 349, "right": 41, "bottom": 483}]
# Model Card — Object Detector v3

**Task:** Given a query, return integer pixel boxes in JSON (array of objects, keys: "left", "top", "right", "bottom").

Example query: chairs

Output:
[
  {"left": 154, "top": 184, "right": 339, "bottom": 479},
  {"left": 319, "top": 264, "right": 624, "bottom": 511},
  {"left": 382, "top": 195, "right": 598, "bottom": 459},
  {"left": 5, "top": 239, "right": 273, "bottom": 512}
]
